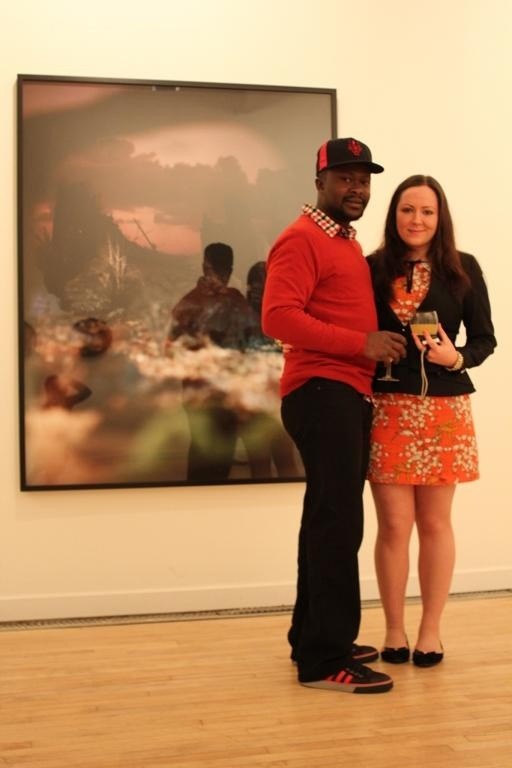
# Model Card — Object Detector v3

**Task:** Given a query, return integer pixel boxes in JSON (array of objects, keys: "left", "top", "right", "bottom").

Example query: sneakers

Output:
[{"left": 291, "top": 643, "right": 393, "bottom": 693}]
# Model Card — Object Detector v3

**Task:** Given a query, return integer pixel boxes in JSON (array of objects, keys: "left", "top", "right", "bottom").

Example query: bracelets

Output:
[{"left": 448, "top": 353, "right": 463, "bottom": 372}]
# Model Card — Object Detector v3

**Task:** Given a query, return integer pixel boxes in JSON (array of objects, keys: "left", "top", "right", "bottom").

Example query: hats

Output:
[{"left": 316, "top": 138, "right": 384, "bottom": 174}]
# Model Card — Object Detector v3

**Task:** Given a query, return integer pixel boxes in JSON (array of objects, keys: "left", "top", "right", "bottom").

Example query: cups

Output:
[{"left": 410, "top": 310, "right": 439, "bottom": 339}]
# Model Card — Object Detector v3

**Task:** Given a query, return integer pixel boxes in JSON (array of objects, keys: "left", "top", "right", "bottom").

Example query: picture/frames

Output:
[{"left": 16, "top": 72, "right": 340, "bottom": 492}]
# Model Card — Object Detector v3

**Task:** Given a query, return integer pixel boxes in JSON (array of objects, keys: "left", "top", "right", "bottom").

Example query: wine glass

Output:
[{"left": 377, "top": 358, "right": 400, "bottom": 383}]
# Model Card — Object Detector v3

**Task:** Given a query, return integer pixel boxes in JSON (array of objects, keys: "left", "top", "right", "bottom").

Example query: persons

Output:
[
  {"left": 365, "top": 174, "right": 499, "bottom": 670},
  {"left": 262, "top": 137, "right": 407, "bottom": 691},
  {"left": 169, "top": 242, "right": 300, "bottom": 481}
]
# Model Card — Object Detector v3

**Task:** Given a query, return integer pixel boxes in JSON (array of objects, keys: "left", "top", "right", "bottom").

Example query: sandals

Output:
[{"left": 381, "top": 634, "right": 445, "bottom": 667}]
